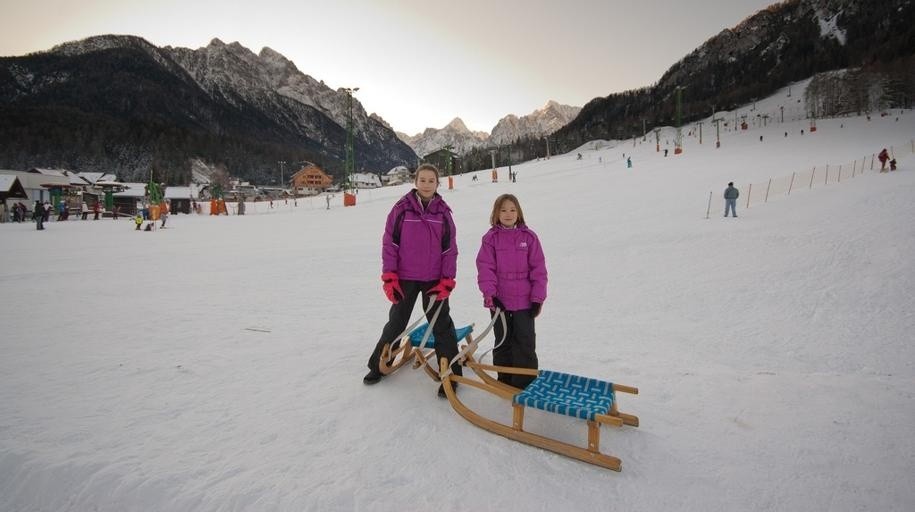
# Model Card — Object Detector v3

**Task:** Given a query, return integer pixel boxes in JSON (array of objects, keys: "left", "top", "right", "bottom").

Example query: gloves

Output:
[
  {"left": 529, "top": 303, "right": 540, "bottom": 318},
  {"left": 489, "top": 297, "right": 505, "bottom": 313},
  {"left": 426, "top": 279, "right": 456, "bottom": 300},
  {"left": 381, "top": 273, "right": 405, "bottom": 304}
]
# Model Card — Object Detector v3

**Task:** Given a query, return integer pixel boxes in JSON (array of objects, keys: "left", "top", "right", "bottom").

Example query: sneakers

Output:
[
  {"left": 363, "top": 370, "right": 381, "bottom": 384},
  {"left": 438, "top": 381, "right": 456, "bottom": 397}
]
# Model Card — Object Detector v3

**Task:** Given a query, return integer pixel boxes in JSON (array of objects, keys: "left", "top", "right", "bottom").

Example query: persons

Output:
[
  {"left": 664, "top": 149, "right": 668, "bottom": 157},
  {"left": 134, "top": 200, "right": 168, "bottom": 230},
  {"left": 878, "top": 149, "right": 897, "bottom": 172},
  {"left": 492, "top": 168, "right": 498, "bottom": 183},
  {"left": 80, "top": 200, "right": 121, "bottom": 220},
  {"left": 476, "top": 195, "right": 548, "bottom": 389},
  {"left": 723, "top": 182, "right": 738, "bottom": 217},
  {"left": 513, "top": 171, "right": 518, "bottom": 182},
  {"left": 577, "top": 153, "right": 582, "bottom": 160},
  {"left": 628, "top": 156, "right": 632, "bottom": 168},
  {"left": 237, "top": 197, "right": 245, "bottom": 215},
  {"left": 9, "top": 200, "right": 71, "bottom": 230},
  {"left": 363, "top": 163, "right": 459, "bottom": 400}
]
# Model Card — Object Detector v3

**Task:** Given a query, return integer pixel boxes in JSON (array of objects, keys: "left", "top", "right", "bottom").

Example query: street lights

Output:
[
  {"left": 673, "top": 86, "right": 685, "bottom": 155},
  {"left": 443, "top": 143, "right": 455, "bottom": 190},
  {"left": 337, "top": 86, "right": 360, "bottom": 206},
  {"left": 278, "top": 160, "right": 286, "bottom": 189}
]
